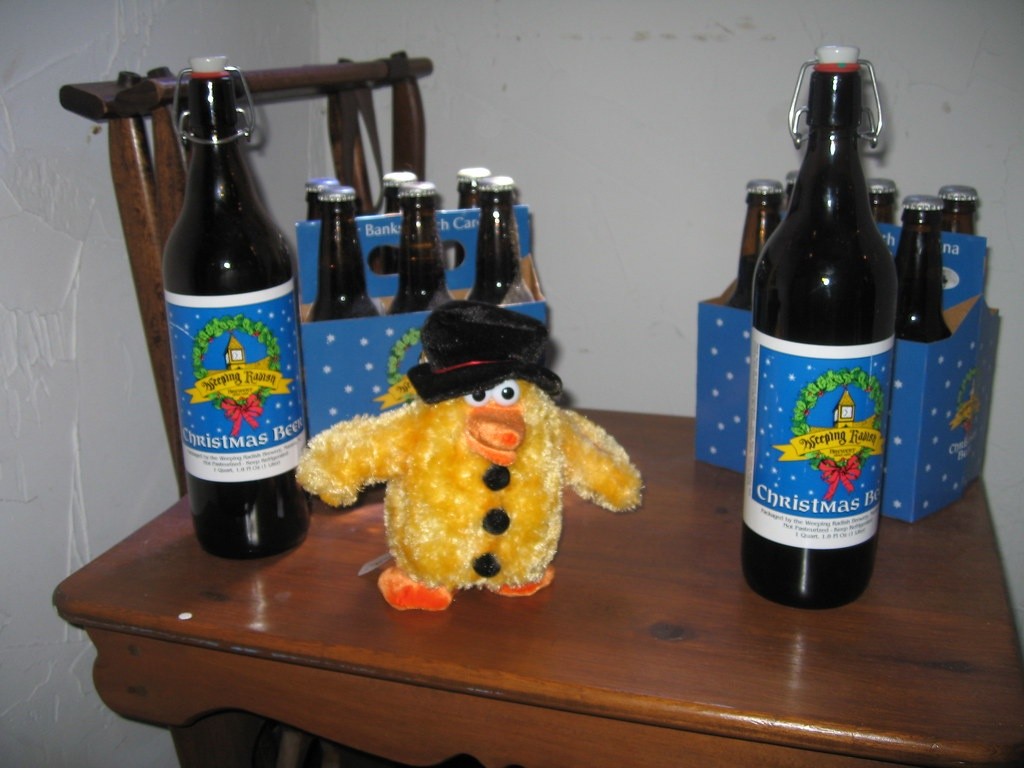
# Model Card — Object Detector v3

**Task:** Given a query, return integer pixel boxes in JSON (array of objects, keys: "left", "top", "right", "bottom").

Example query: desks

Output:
[{"left": 52, "top": 406, "right": 1024, "bottom": 768}]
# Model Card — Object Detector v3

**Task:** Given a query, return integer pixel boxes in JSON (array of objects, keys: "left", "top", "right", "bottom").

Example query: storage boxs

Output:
[
  {"left": 693, "top": 221, "right": 1004, "bottom": 522},
  {"left": 294, "top": 203, "right": 551, "bottom": 436}
]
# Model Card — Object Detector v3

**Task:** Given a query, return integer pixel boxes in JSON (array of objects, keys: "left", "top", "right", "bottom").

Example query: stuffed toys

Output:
[{"left": 295, "top": 299, "right": 645, "bottom": 611}]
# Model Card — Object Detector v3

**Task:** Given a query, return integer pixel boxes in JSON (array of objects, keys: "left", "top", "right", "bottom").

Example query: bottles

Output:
[
  {"left": 939, "top": 185, "right": 978, "bottom": 235},
  {"left": 786, "top": 171, "right": 798, "bottom": 208},
  {"left": 895, "top": 195, "right": 952, "bottom": 343},
  {"left": 386, "top": 181, "right": 454, "bottom": 316},
  {"left": 305, "top": 179, "right": 340, "bottom": 221},
  {"left": 457, "top": 167, "right": 491, "bottom": 211},
  {"left": 162, "top": 57, "right": 311, "bottom": 559},
  {"left": 383, "top": 172, "right": 416, "bottom": 214},
  {"left": 866, "top": 178, "right": 897, "bottom": 225},
  {"left": 742, "top": 46, "right": 897, "bottom": 610},
  {"left": 308, "top": 185, "right": 380, "bottom": 323},
  {"left": 727, "top": 180, "right": 783, "bottom": 311},
  {"left": 468, "top": 176, "right": 536, "bottom": 305}
]
